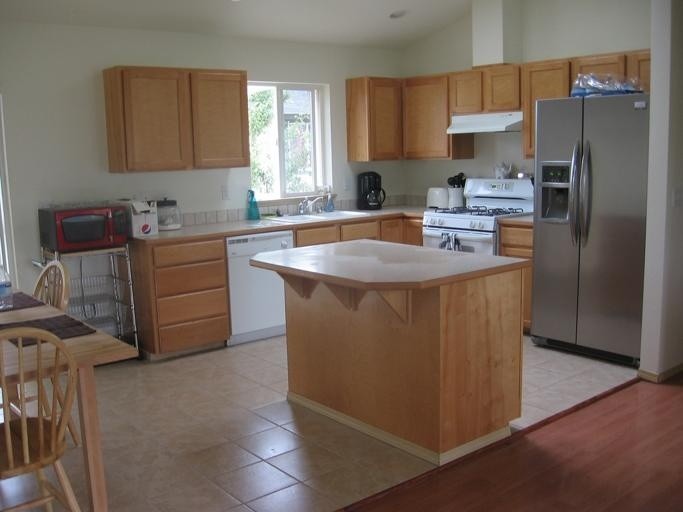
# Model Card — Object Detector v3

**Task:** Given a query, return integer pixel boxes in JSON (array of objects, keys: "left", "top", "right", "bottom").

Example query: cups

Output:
[{"left": 447, "top": 188, "right": 464, "bottom": 210}]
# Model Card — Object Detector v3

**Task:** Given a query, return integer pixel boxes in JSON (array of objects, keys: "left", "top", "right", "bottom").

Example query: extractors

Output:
[{"left": 445, "top": 112, "right": 523, "bottom": 136}]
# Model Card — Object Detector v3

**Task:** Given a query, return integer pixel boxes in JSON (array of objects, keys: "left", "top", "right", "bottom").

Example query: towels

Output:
[{"left": 438, "top": 231, "right": 461, "bottom": 251}]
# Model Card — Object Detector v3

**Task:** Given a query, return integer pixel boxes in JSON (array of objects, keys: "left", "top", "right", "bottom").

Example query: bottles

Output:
[
  {"left": 156, "top": 197, "right": 181, "bottom": 231},
  {"left": 324, "top": 192, "right": 334, "bottom": 213},
  {"left": 302, "top": 196, "right": 311, "bottom": 212},
  {"left": 0, "top": 265, "right": 12, "bottom": 311}
]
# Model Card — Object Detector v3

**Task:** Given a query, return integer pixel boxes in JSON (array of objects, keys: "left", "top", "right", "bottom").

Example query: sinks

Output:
[
  {"left": 316, "top": 211, "right": 367, "bottom": 218},
  {"left": 266, "top": 215, "right": 324, "bottom": 223}
]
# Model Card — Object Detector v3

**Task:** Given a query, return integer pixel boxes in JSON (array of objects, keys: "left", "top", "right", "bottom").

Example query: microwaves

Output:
[{"left": 38, "top": 205, "right": 127, "bottom": 251}]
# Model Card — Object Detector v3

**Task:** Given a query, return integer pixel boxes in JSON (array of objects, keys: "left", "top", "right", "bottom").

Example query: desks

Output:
[{"left": 251, "top": 236, "right": 535, "bottom": 471}]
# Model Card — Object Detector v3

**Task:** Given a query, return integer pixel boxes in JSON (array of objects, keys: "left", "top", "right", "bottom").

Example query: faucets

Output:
[{"left": 297, "top": 196, "right": 324, "bottom": 215}]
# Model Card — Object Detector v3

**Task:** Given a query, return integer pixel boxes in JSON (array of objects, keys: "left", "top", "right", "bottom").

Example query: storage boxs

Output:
[{"left": 113, "top": 196, "right": 160, "bottom": 239}]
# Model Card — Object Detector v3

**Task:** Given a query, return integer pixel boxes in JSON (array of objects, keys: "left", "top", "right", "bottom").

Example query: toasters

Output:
[{"left": 424, "top": 187, "right": 448, "bottom": 209}]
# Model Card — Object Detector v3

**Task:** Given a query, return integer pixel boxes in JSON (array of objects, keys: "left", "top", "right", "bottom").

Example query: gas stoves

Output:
[{"left": 420, "top": 204, "right": 524, "bottom": 232}]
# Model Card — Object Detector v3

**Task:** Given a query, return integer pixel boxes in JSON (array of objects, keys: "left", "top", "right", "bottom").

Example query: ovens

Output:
[{"left": 420, "top": 227, "right": 498, "bottom": 257}]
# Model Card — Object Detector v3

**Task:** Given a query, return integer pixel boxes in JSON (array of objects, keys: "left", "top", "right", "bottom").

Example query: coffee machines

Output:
[{"left": 357, "top": 172, "right": 382, "bottom": 210}]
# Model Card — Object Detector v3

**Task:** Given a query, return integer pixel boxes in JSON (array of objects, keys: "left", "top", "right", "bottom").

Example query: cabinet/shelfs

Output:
[
  {"left": 568, "top": 50, "right": 626, "bottom": 93},
  {"left": 343, "top": 76, "right": 402, "bottom": 165},
  {"left": 496, "top": 216, "right": 534, "bottom": 336},
  {"left": 519, "top": 57, "right": 570, "bottom": 160},
  {"left": 116, "top": 234, "right": 233, "bottom": 364},
  {"left": 37, "top": 245, "right": 140, "bottom": 357},
  {"left": 337, "top": 216, "right": 382, "bottom": 243},
  {"left": 399, "top": 72, "right": 475, "bottom": 162},
  {"left": 101, "top": 62, "right": 251, "bottom": 175},
  {"left": 382, "top": 213, "right": 403, "bottom": 244},
  {"left": 625, "top": 46, "right": 650, "bottom": 94},
  {"left": 402, "top": 211, "right": 424, "bottom": 247},
  {"left": 448, "top": 65, "right": 522, "bottom": 117},
  {"left": 293, "top": 220, "right": 342, "bottom": 249}
]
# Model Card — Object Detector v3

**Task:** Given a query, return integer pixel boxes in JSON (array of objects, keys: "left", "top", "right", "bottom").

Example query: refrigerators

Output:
[{"left": 531, "top": 92, "right": 648, "bottom": 367}]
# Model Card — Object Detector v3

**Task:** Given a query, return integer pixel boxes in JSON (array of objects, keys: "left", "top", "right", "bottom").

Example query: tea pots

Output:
[{"left": 494, "top": 160, "right": 513, "bottom": 179}]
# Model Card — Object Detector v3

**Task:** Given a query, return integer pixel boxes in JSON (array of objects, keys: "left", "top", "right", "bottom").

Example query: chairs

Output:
[
  {"left": 0, "top": 256, "right": 82, "bottom": 455},
  {"left": 0, "top": 325, "right": 86, "bottom": 512}
]
